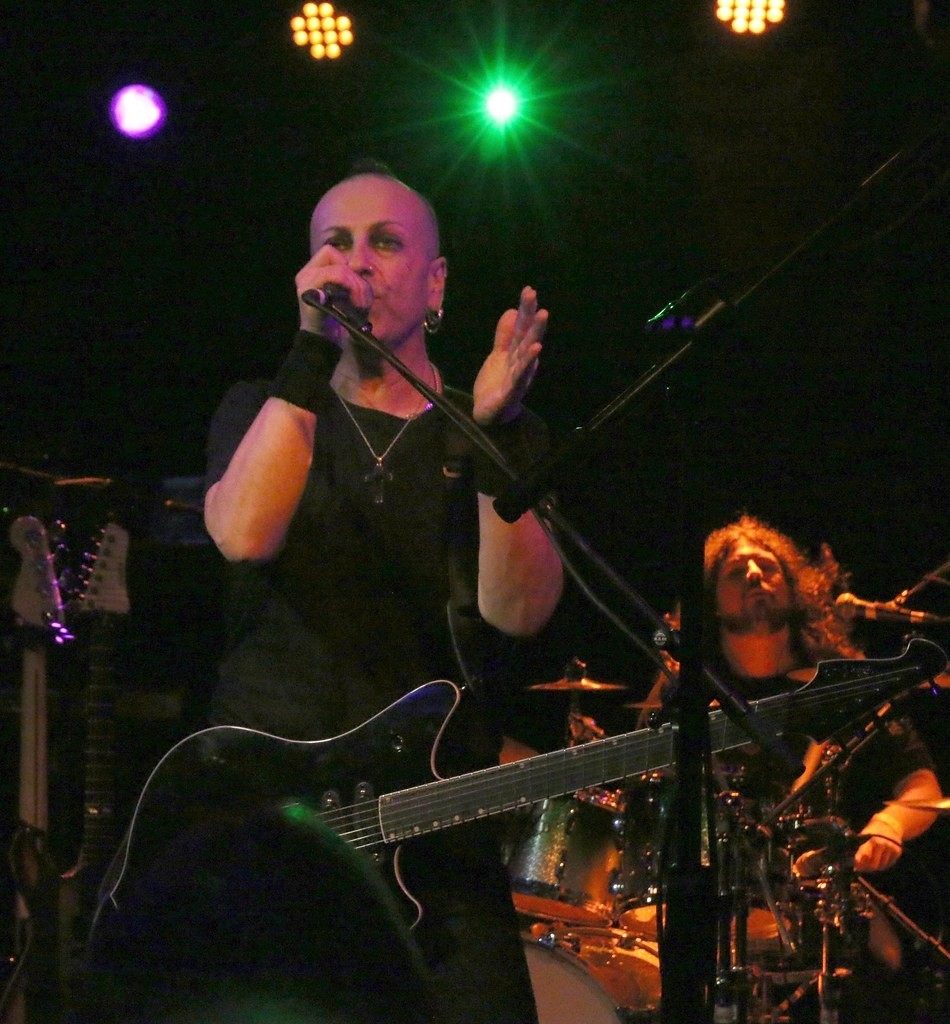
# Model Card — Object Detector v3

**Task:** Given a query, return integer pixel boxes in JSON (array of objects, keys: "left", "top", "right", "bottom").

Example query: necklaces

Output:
[{"left": 326, "top": 359, "right": 441, "bottom": 477}]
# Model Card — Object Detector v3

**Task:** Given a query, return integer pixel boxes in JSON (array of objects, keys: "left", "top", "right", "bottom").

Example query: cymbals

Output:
[{"left": 509, "top": 672, "right": 631, "bottom": 692}]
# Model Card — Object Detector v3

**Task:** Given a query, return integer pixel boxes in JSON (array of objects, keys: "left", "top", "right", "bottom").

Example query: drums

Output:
[
  {"left": 613, "top": 765, "right": 795, "bottom": 940},
  {"left": 514, "top": 923, "right": 661, "bottom": 1023},
  {"left": 497, "top": 782, "right": 630, "bottom": 926}
]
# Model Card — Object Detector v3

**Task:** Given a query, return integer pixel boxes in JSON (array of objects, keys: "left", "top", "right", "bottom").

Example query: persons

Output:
[
  {"left": 615, "top": 512, "right": 944, "bottom": 1024},
  {"left": 204, "top": 155, "right": 566, "bottom": 1024}
]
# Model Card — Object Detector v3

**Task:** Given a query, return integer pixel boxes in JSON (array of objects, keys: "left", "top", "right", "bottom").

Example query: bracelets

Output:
[
  {"left": 471, "top": 405, "right": 550, "bottom": 499},
  {"left": 267, "top": 328, "right": 344, "bottom": 415}
]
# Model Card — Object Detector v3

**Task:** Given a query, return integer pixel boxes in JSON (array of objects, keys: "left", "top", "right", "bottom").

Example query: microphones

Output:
[
  {"left": 833, "top": 591, "right": 942, "bottom": 628},
  {"left": 318, "top": 285, "right": 351, "bottom": 307}
]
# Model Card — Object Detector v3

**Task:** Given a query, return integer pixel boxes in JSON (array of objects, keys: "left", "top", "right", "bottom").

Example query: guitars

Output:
[
  {"left": 103, "top": 637, "right": 950, "bottom": 944},
  {"left": 1, "top": 511, "right": 140, "bottom": 1024}
]
